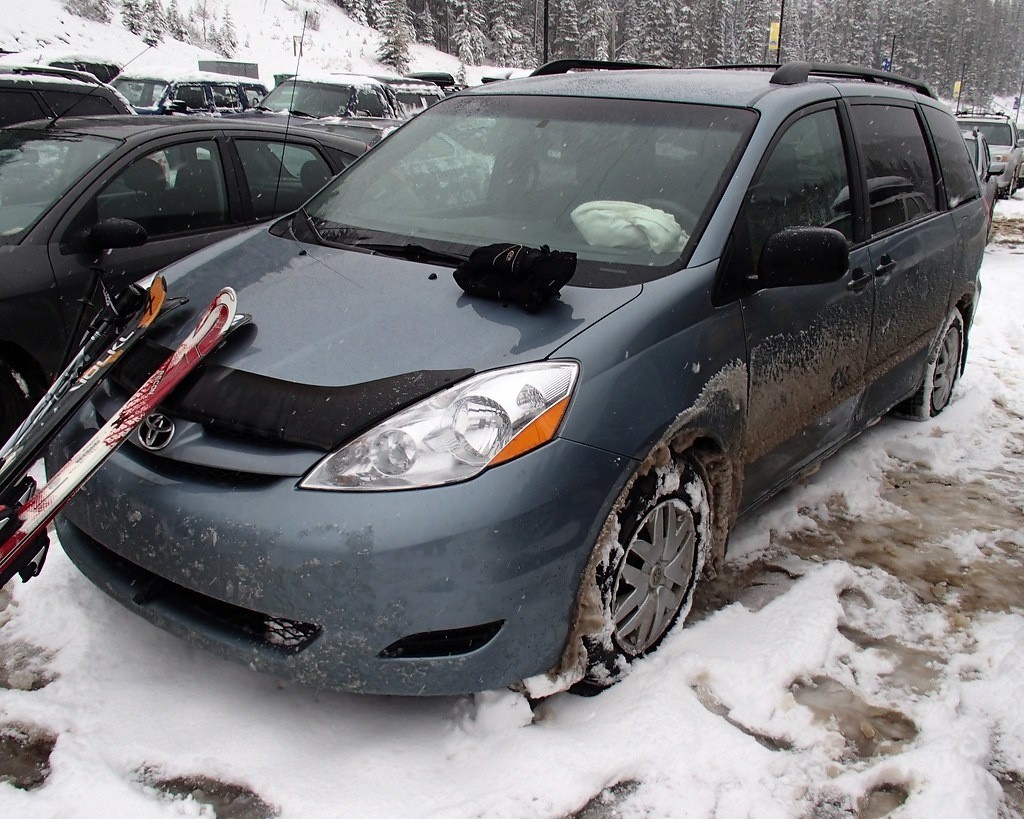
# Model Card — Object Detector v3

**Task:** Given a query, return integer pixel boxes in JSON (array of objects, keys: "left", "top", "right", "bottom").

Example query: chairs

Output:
[
  {"left": 180, "top": 160, "right": 218, "bottom": 211},
  {"left": 124, "top": 159, "right": 167, "bottom": 218},
  {"left": 292, "top": 160, "right": 332, "bottom": 209}
]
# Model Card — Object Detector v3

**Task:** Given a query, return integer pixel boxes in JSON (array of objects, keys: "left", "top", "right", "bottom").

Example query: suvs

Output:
[
  {"left": 954, "top": 111, "right": 1024, "bottom": 198},
  {"left": 959, "top": 124, "right": 1007, "bottom": 230},
  {"left": 39, "top": 58, "right": 989, "bottom": 694}
]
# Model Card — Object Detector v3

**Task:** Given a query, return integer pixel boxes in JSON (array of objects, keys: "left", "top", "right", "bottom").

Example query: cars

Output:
[
  {"left": 1014, "top": 124, "right": 1024, "bottom": 186},
  {"left": 273, "top": 115, "right": 498, "bottom": 219},
  {"left": 0, "top": 59, "right": 512, "bottom": 131},
  {"left": 0, "top": 113, "right": 376, "bottom": 449}
]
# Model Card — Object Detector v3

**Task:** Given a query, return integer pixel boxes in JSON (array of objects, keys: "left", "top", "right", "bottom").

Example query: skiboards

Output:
[{"left": 0, "top": 270, "right": 252, "bottom": 590}]
